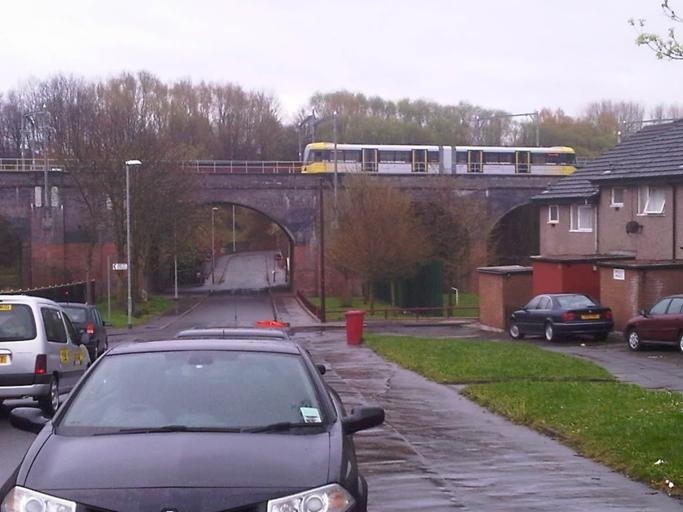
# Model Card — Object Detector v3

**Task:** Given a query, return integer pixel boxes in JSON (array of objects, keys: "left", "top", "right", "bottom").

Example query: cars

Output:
[
  {"left": 622, "top": 294, "right": 683, "bottom": 354},
  {"left": 0, "top": 337, "right": 386, "bottom": 511},
  {"left": 161, "top": 326, "right": 326, "bottom": 375},
  {"left": 508, "top": 293, "right": 614, "bottom": 342}
]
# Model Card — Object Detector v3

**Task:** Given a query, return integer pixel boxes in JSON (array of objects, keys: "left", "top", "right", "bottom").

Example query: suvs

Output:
[{"left": 55, "top": 300, "right": 112, "bottom": 363}]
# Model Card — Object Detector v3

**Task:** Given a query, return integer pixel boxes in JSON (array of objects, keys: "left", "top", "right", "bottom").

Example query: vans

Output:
[{"left": 0, "top": 295, "right": 90, "bottom": 417}]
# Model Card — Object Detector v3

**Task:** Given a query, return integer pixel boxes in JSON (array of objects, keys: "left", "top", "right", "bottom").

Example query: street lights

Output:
[
  {"left": 211, "top": 207, "right": 219, "bottom": 286},
  {"left": 125, "top": 160, "right": 142, "bottom": 331}
]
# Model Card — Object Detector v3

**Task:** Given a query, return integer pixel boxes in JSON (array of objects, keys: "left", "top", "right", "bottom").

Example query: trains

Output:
[{"left": 300, "top": 140, "right": 578, "bottom": 176}]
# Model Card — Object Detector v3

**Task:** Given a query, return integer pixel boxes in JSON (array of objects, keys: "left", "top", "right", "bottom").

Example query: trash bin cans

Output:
[{"left": 344, "top": 310, "right": 366, "bottom": 345}]
